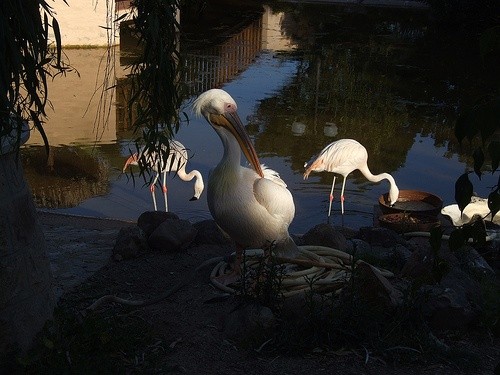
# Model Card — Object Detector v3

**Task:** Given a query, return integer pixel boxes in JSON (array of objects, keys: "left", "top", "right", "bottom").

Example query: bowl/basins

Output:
[
  {"left": 378, "top": 213, "right": 442, "bottom": 235},
  {"left": 377, "top": 189, "right": 444, "bottom": 216}
]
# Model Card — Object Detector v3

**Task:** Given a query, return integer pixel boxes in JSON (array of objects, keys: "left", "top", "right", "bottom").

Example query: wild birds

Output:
[
  {"left": 454, "top": 170, "right": 500, "bottom": 222},
  {"left": 122, "top": 139, "right": 204, "bottom": 212},
  {"left": 303, "top": 138, "right": 400, "bottom": 228},
  {"left": 190, "top": 89, "right": 296, "bottom": 278}
]
